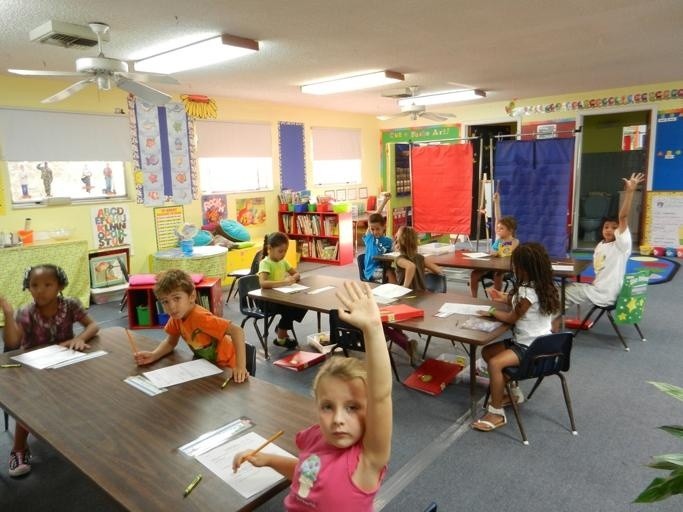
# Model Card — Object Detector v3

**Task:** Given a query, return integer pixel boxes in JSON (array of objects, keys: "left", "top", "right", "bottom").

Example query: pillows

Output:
[{"left": 193, "top": 219, "right": 256, "bottom": 253}]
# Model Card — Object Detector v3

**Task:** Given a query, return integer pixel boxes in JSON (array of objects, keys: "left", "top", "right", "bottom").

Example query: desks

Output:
[{"left": 352, "top": 210, "right": 386, "bottom": 252}]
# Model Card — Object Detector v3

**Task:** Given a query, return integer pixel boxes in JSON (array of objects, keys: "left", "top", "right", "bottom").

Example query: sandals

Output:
[
  {"left": 472, "top": 405, "right": 507, "bottom": 431},
  {"left": 501, "top": 386, "right": 524, "bottom": 407}
]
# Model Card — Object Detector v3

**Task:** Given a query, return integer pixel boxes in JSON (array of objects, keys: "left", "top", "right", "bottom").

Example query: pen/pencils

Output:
[
  {"left": 220, "top": 378, "right": 232, "bottom": 388},
  {"left": 183, "top": 474, "right": 203, "bottom": 496},
  {"left": 1, "top": 364, "right": 22, "bottom": 368},
  {"left": 125, "top": 327, "right": 137, "bottom": 353},
  {"left": 240, "top": 431, "right": 283, "bottom": 464},
  {"left": 496, "top": 179, "right": 500, "bottom": 193}
]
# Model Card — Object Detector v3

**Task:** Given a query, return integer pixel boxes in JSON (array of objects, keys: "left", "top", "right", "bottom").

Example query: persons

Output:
[
  {"left": 102, "top": 162, "right": 114, "bottom": 194},
  {"left": 35, "top": 161, "right": 56, "bottom": 199},
  {"left": 257, "top": 232, "right": 300, "bottom": 348},
  {"left": 469, "top": 191, "right": 519, "bottom": 299},
  {"left": 231, "top": 279, "right": 394, "bottom": 512},
  {"left": 79, "top": 162, "right": 95, "bottom": 195},
  {"left": 363, "top": 192, "right": 392, "bottom": 283},
  {"left": 381, "top": 226, "right": 444, "bottom": 367},
  {"left": 0, "top": 265, "right": 100, "bottom": 478},
  {"left": 132, "top": 267, "right": 250, "bottom": 384},
  {"left": 15, "top": 162, "right": 31, "bottom": 200},
  {"left": 470, "top": 241, "right": 562, "bottom": 431},
  {"left": 550, "top": 169, "right": 647, "bottom": 335}
]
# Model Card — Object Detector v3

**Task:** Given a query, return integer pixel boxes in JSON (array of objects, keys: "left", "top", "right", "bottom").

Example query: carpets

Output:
[{"left": 565, "top": 251, "right": 682, "bottom": 283}]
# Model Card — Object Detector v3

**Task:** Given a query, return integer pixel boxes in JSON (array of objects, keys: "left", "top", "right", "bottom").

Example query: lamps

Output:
[
  {"left": 300, "top": 70, "right": 404, "bottom": 96},
  {"left": 398, "top": 90, "right": 487, "bottom": 107},
  {"left": 92, "top": 71, "right": 111, "bottom": 92},
  {"left": 134, "top": 34, "right": 260, "bottom": 75}
]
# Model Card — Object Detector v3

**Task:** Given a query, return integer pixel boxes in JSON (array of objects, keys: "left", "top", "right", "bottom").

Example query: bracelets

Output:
[{"left": 489, "top": 307, "right": 496, "bottom": 317}]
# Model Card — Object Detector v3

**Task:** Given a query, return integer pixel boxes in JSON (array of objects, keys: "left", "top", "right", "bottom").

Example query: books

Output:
[
  {"left": 276, "top": 187, "right": 341, "bottom": 263},
  {"left": 154, "top": 294, "right": 210, "bottom": 314}
]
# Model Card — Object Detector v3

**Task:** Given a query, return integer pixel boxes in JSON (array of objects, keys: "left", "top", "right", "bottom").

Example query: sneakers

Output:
[
  {"left": 409, "top": 339, "right": 419, "bottom": 367},
  {"left": 273, "top": 338, "right": 297, "bottom": 348},
  {"left": 8, "top": 448, "right": 32, "bottom": 476}
]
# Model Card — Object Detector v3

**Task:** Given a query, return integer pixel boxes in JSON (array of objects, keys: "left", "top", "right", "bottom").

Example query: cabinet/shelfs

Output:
[{"left": 278, "top": 210, "right": 353, "bottom": 266}]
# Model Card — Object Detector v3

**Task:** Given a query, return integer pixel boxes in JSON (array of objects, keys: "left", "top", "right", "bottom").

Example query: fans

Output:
[
  {"left": 376, "top": 86, "right": 455, "bottom": 121},
  {"left": 7, "top": 22, "right": 179, "bottom": 106}
]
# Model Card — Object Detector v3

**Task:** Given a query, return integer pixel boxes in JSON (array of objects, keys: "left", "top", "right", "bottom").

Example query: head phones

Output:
[{"left": 22, "top": 264, "right": 68, "bottom": 290}]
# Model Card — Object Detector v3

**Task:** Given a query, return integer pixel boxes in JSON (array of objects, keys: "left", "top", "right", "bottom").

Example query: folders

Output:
[{"left": 563, "top": 317, "right": 593, "bottom": 330}]
